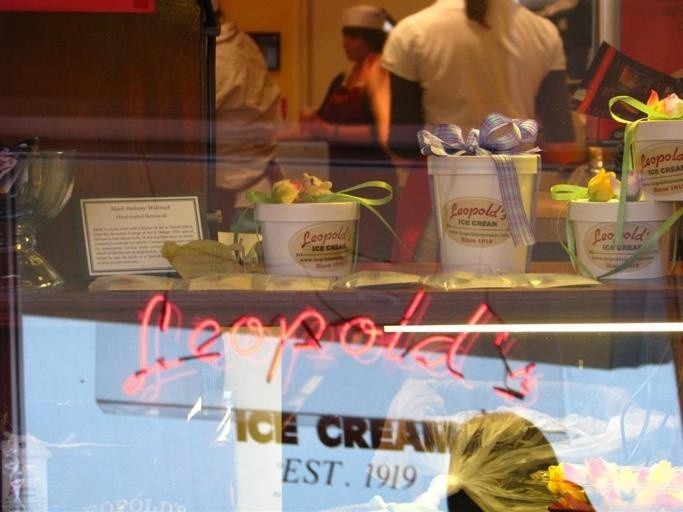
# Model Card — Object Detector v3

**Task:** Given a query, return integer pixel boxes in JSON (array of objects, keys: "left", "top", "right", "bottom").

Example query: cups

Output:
[
  {"left": 428, "top": 155, "right": 537, "bottom": 274},
  {"left": 630, "top": 120, "right": 683, "bottom": 202},
  {"left": 566, "top": 199, "right": 674, "bottom": 280},
  {"left": 253, "top": 200, "right": 360, "bottom": 278}
]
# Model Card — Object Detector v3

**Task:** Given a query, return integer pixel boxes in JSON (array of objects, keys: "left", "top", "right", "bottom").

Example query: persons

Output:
[
  {"left": 377, "top": 0, "right": 574, "bottom": 265},
  {"left": 300, "top": 5, "right": 398, "bottom": 264},
  {"left": 212, "top": 0, "right": 286, "bottom": 233}
]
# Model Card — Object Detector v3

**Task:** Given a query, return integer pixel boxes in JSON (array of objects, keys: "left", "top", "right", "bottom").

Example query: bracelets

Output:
[{"left": 333, "top": 124, "right": 339, "bottom": 143}]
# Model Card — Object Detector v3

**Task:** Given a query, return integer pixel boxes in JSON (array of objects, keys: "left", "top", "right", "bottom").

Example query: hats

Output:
[{"left": 341, "top": 5, "right": 385, "bottom": 30}]
